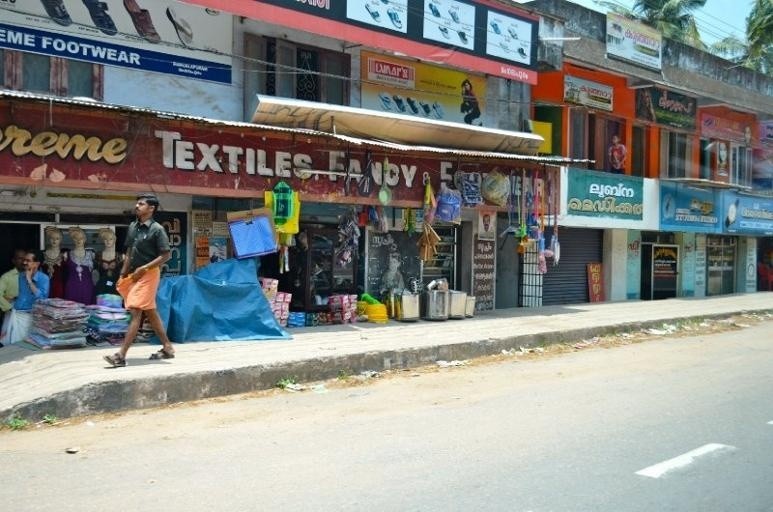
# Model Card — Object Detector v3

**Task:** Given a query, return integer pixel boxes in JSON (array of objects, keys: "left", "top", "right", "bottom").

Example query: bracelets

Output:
[
  {"left": 142, "top": 265, "right": 149, "bottom": 271},
  {"left": 120, "top": 272, "right": 124, "bottom": 276}
]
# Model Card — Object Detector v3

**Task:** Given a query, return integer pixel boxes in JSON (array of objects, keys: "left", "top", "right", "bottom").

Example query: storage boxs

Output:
[{"left": 258, "top": 277, "right": 358, "bottom": 328}]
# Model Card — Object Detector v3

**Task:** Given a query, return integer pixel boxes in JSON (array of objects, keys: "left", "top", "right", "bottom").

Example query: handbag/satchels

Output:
[{"left": 227, "top": 208, "right": 277, "bottom": 259}]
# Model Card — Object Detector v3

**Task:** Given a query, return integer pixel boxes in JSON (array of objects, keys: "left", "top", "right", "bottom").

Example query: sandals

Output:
[
  {"left": 103, "top": 353, "right": 125, "bottom": 367},
  {"left": 149, "top": 351, "right": 174, "bottom": 361}
]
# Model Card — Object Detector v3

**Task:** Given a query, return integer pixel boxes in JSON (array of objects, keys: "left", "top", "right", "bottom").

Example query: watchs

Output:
[{"left": 725, "top": 198, "right": 740, "bottom": 228}]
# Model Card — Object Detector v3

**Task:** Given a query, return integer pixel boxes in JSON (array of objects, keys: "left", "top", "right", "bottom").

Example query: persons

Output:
[
  {"left": 479, "top": 214, "right": 495, "bottom": 240},
  {"left": 380, "top": 254, "right": 406, "bottom": 295},
  {"left": 718, "top": 141, "right": 730, "bottom": 174},
  {"left": 0, "top": 246, "right": 28, "bottom": 350},
  {"left": 102, "top": 194, "right": 176, "bottom": 367},
  {"left": 92, "top": 228, "right": 126, "bottom": 298},
  {"left": 38, "top": 227, "right": 66, "bottom": 300},
  {"left": 4, "top": 248, "right": 50, "bottom": 345},
  {"left": 460, "top": 78, "right": 481, "bottom": 124},
  {"left": 607, "top": 133, "right": 628, "bottom": 175},
  {"left": 743, "top": 125, "right": 752, "bottom": 147},
  {"left": 60, "top": 226, "right": 97, "bottom": 306}
]
